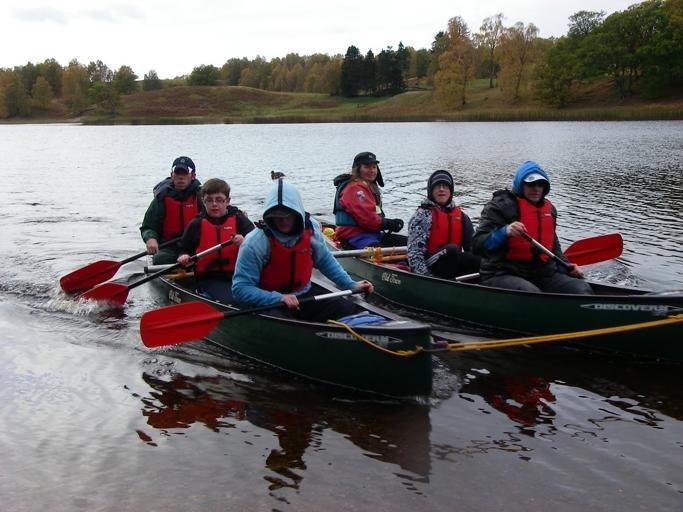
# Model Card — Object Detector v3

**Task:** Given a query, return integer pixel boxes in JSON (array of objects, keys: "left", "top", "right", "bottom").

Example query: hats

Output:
[
  {"left": 523, "top": 173, "right": 550, "bottom": 186},
  {"left": 265, "top": 210, "right": 294, "bottom": 218},
  {"left": 431, "top": 172, "right": 452, "bottom": 189},
  {"left": 171, "top": 156, "right": 195, "bottom": 174},
  {"left": 354, "top": 152, "right": 380, "bottom": 165}
]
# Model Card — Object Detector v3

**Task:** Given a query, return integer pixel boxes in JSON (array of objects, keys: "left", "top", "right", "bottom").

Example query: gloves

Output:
[{"left": 381, "top": 218, "right": 404, "bottom": 233}]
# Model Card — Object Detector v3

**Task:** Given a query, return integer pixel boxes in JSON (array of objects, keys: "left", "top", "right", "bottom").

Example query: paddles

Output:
[
  {"left": 453, "top": 232, "right": 623, "bottom": 282},
  {"left": 60, "top": 235, "right": 182, "bottom": 293},
  {"left": 73, "top": 239, "right": 234, "bottom": 306},
  {"left": 139, "top": 286, "right": 366, "bottom": 348}
]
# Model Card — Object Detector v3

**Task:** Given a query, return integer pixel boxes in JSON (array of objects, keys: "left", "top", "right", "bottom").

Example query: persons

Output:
[
  {"left": 407, "top": 170, "right": 474, "bottom": 280},
  {"left": 140, "top": 156, "right": 202, "bottom": 266},
  {"left": 333, "top": 152, "right": 403, "bottom": 248},
  {"left": 472, "top": 161, "right": 593, "bottom": 295},
  {"left": 231, "top": 179, "right": 373, "bottom": 317},
  {"left": 177, "top": 178, "right": 254, "bottom": 306}
]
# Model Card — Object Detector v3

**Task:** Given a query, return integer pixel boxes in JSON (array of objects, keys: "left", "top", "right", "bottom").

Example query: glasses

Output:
[{"left": 205, "top": 197, "right": 227, "bottom": 204}]
